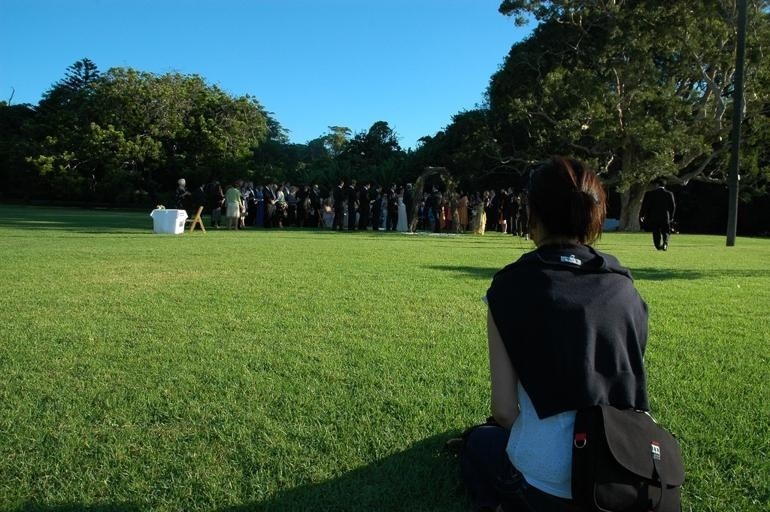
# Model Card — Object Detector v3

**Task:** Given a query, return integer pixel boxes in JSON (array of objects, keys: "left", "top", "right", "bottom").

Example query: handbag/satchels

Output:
[{"left": 571, "top": 403, "right": 685, "bottom": 512}]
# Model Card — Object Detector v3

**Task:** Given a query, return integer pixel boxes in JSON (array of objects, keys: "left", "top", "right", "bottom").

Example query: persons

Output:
[
  {"left": 639, "top": 178, "right": 676, "bottom": 250},
  {"left": 460, "top": 156, "right": 648, "bottom": 512},
  {"left": 175, "top": 179, "right": 191, "bottom": 210}
]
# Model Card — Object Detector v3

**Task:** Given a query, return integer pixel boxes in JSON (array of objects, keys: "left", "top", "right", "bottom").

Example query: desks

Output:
[{"left": 150, "top": 208, "right": 187, "bottom": 234}]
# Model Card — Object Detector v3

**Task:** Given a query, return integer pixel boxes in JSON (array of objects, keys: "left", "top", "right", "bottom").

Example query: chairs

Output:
[{"left": 185, "top": 206, "right": 207, "bottom": 234}]
